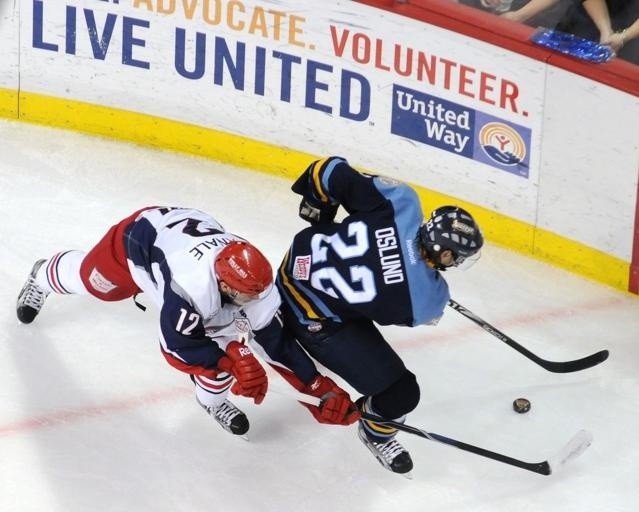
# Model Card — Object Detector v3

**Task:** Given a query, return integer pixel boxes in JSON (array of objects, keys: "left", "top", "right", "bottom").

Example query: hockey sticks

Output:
[
  {"left": 267, "top": 384, "right": 591, "bottom": 474},
  {"left": 446, "top": 299, "right": 609, "bottom": 373}
]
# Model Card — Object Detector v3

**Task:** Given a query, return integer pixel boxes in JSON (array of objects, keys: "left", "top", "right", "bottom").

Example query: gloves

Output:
[
  {"left": 296, "top": 194, "right": 341, "bottom": 225},
  {"left": 219, "top": 339, "right": 269, "bottom": 405},
  {"left": 299, "top": 374, "right": 364, "bottom": 426}
]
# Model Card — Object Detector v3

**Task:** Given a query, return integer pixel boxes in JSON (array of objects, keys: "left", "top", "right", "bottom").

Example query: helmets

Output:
[
  {"left": 215, "top": 239, "right": 274, "bottom": 295},
  {"left": 421, "top": 204, "right": 484, "bottom": 258}
]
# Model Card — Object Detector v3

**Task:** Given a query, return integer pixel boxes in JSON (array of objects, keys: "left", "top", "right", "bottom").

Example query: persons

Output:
[
  {"left": 15, "top": 204, "right": 363, "bottom": 437},
  {"left": 275, "top": 154, "right": 483, "bottom": 474},
  {"left": 453, "top": 0, "right": 639, "bottom": 67}
]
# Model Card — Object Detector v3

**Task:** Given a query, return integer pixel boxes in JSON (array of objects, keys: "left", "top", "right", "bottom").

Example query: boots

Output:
[
  {"left": 354, "top": 395, "right": 415, "bottom": 474},
  {"left": 15, "top": 257, "right": 51, "bottom": 324},
  {"left": 194, "top": 393, "right": 250, "bottom": 436}
]
